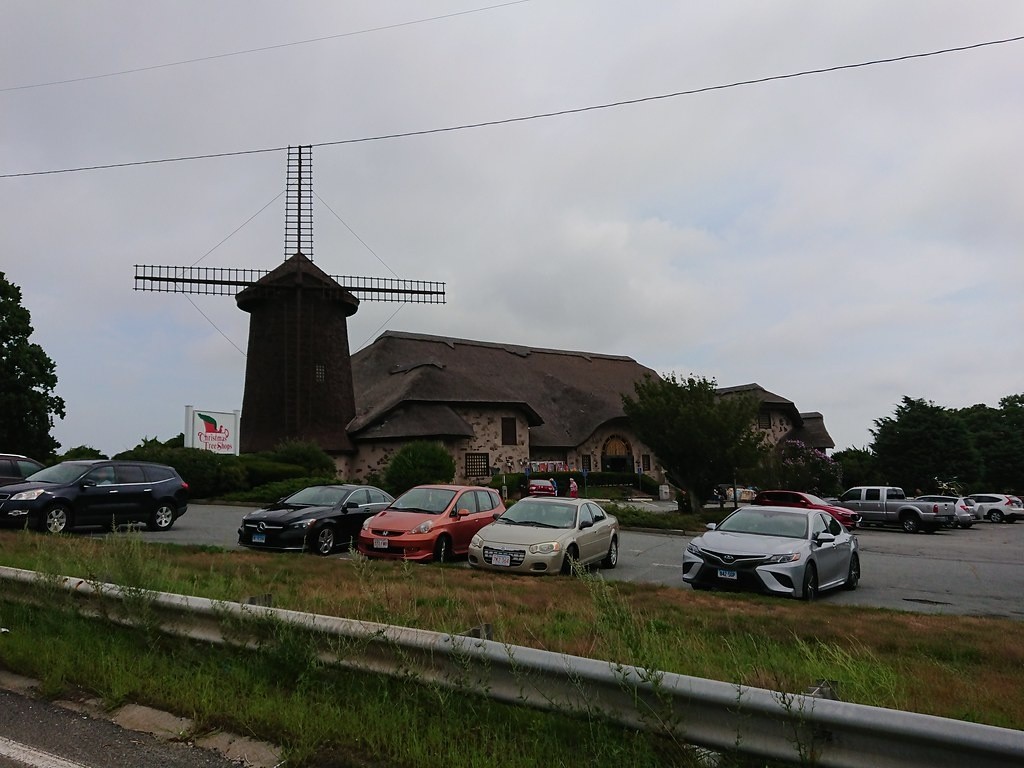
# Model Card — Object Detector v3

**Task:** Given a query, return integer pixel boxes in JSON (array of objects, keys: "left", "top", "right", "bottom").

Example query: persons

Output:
[
  {"left": 550, "top": 478, "right": 557, "bottom": 496},
  {"left": 570, "top": 478, "right": 577, "bottom": 498},
  {"left": 92, "top": 468, "right": 112, "bottom": 485}
]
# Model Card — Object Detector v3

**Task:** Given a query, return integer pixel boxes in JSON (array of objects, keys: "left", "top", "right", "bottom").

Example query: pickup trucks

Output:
[{"left": 823, "top": 485, "right": 958, "bottom": 535}]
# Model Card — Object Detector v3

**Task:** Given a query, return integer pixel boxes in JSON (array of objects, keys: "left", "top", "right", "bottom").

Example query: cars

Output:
[
  {"left": 520, "top": 479, "right": 555, "bottom": 499},
  {"left": 237, "top": 484, "right": 402, "bottom": 558},
  {"left": 358, "top": 484, "right": 507, "bottom": 566},
  {"left": 468, "top": 495, "right": 621, "bottom": 580},
  {"left": 680, "top": 505, "right": 861, "bottom": 602},
  {"left": 750, "top": 491, "right": 861, "bottom": 530}
]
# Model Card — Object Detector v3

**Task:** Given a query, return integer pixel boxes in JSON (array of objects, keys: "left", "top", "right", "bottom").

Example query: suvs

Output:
[
  {"left": 911, "top": 496, "right": 985, "bottom": 529},
  {"left": 0, "top": 454, "right": 63, "bottom": 486},
  {"left": 964, "top": 494, "right": 1024, "bottom": 524},
  {"left": 0, "top": 460, "right": 189, "bottom": 536}
]
